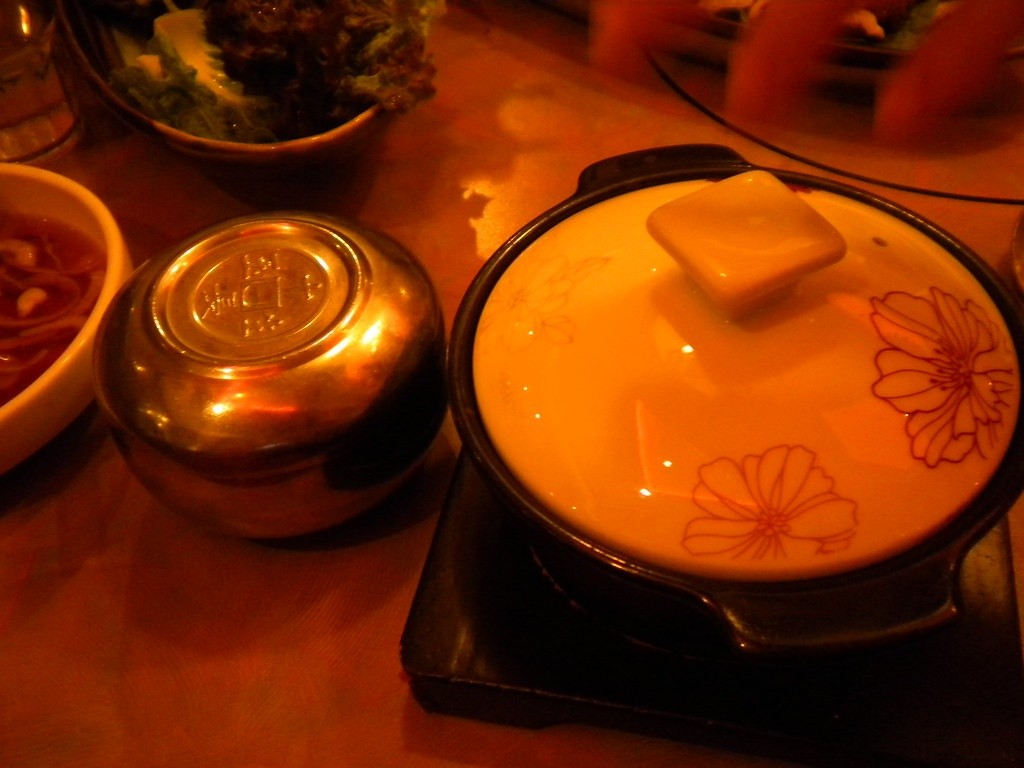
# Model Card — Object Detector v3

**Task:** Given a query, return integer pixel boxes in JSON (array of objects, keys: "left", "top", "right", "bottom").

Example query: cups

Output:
[{"left": 1, "top": 3, "right": 82, "bottom": 172}]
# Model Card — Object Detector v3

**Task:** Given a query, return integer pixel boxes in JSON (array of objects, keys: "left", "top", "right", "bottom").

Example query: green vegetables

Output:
[{"left": 79, "top": 0, "right": 449, "bottom": 142}]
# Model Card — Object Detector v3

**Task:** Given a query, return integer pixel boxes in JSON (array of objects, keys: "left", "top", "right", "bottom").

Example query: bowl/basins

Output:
[
  {"left": 0, "top": 163, "right": 133, "bottom": 479},
  {"left": 90, "top": 208, "right": 447, "bottom": 543},
  {"left": 52, "top": 0, "right": 427, "bottom": 173}
]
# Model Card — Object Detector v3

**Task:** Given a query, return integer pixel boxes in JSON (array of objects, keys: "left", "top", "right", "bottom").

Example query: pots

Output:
[{"left": 444, "top": 142, "right": 1024, "bottom": 658}]
